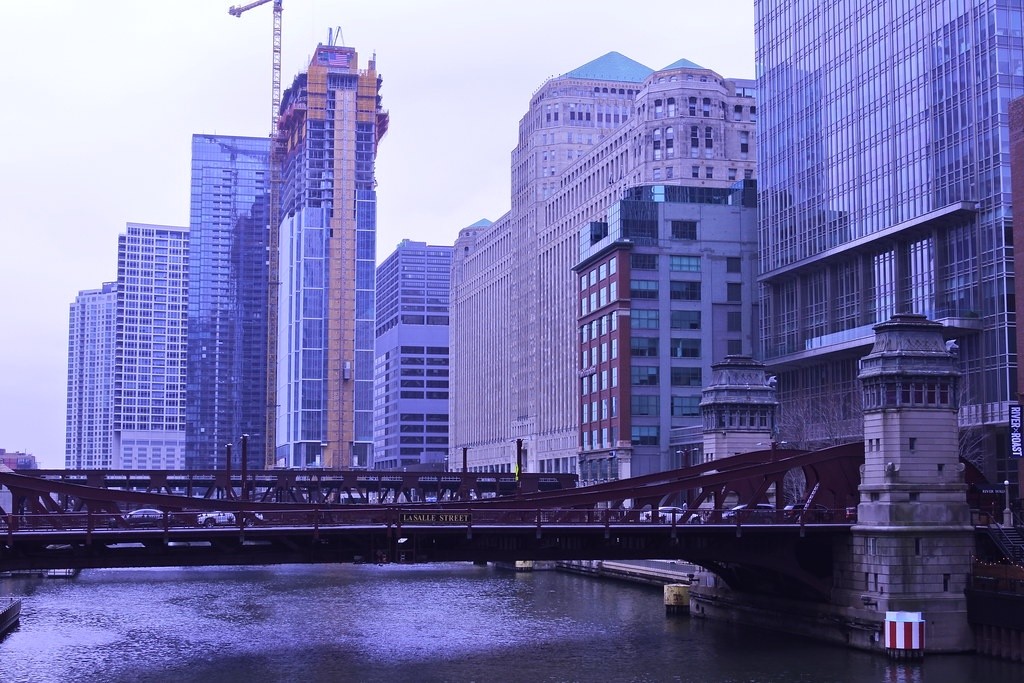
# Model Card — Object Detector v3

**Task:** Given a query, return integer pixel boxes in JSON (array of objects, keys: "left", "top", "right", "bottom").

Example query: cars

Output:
[
  {"left": 121, "top": 508, "right": 176, "bottom": 529},
  {"left": 722, "top": 502, "right": 777, "bottom": 525},
  {"left": 785, "top": 502, "right": 833, "bottom": 526},
  {"left": 644, "top": 506, "right": 705, "bottom": 525},
  {"left": 197, "top": 510, "right": 237, "bottom": 529}
]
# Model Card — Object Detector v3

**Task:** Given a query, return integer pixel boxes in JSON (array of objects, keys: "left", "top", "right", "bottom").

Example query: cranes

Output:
[{"left": 227, "top": 0, "right": 283, "bottom": 472}]
